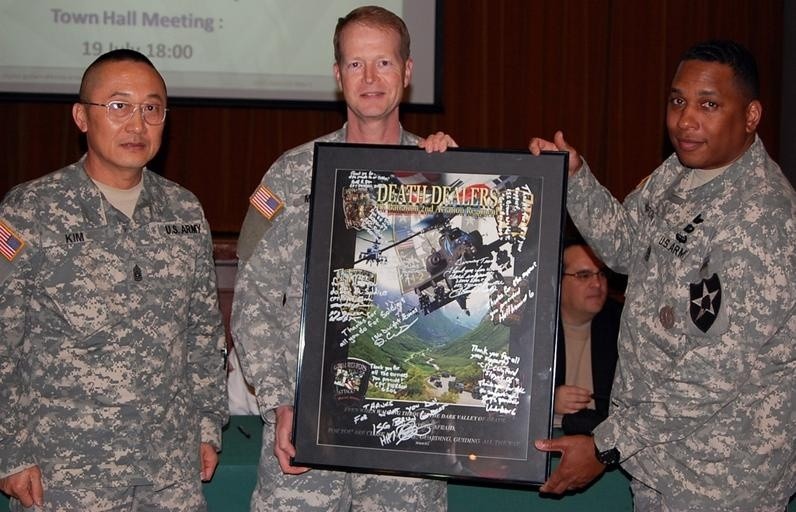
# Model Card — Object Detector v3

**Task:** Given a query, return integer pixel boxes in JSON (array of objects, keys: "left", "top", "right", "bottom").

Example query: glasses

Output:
[
  {"left": 77, "top": 99, "right": 171, "bottom": 126},
  {"left": 564, "top": 265, "right": 610, "bottom": 282}
]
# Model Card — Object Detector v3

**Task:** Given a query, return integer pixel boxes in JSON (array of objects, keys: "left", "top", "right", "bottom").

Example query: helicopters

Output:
[{"left": 350, "top": 194, "right": 519, "bottom": 319}]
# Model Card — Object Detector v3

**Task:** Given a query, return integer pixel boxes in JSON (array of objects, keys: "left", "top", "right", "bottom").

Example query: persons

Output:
[
  {"left": 552, "top": 239, "right": 621, "bottom": 436},
  {"left": 0, "top": 48, "right": 231, "bottom": 512},
  {"left": 231, "top": 7, "right": 465, "bottom": 512},
  {"left": 525, "top": 33, "right": 794, "bottom": 512}
]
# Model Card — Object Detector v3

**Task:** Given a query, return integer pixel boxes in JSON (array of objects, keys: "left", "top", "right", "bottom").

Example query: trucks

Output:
[{"left": 428, "top": 370, "right": 484, "bottom": 401}]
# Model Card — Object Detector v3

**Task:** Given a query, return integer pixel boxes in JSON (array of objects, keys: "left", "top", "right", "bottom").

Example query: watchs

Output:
[{"left": 593, "top": 429, "right": 620, "bottom": 469}]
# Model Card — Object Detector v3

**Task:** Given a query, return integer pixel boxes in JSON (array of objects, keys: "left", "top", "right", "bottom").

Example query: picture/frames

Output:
[{"left": 288, "top": 142, "right": 569, "bottom": 491}]
